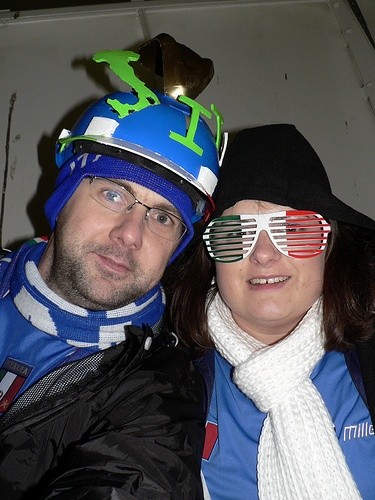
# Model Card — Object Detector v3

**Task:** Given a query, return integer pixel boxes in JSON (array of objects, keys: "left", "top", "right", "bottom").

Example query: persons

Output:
[
  {"left": 0, "top": 93, "right": 219, "bottom": 500},
  {"left": 197, "top": 125, "right": 375, "bottom": 500}
]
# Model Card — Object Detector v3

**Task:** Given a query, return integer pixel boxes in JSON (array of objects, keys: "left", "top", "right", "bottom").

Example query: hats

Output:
[{"left": 207, "top": 123, "right": 335, "bottom": 224}]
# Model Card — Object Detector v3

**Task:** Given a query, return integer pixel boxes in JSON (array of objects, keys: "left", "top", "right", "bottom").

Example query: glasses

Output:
[
  {"left": 83, "top": 172, "right": 188, "bottom": 242},
  {"left": 201, "top": 209, "right": 332, "bottom": 263}
]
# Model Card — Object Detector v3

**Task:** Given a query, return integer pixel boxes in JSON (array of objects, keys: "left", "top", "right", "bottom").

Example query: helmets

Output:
[{"left": 50, "top": 32, "right": 225, "bottom": 225}]
